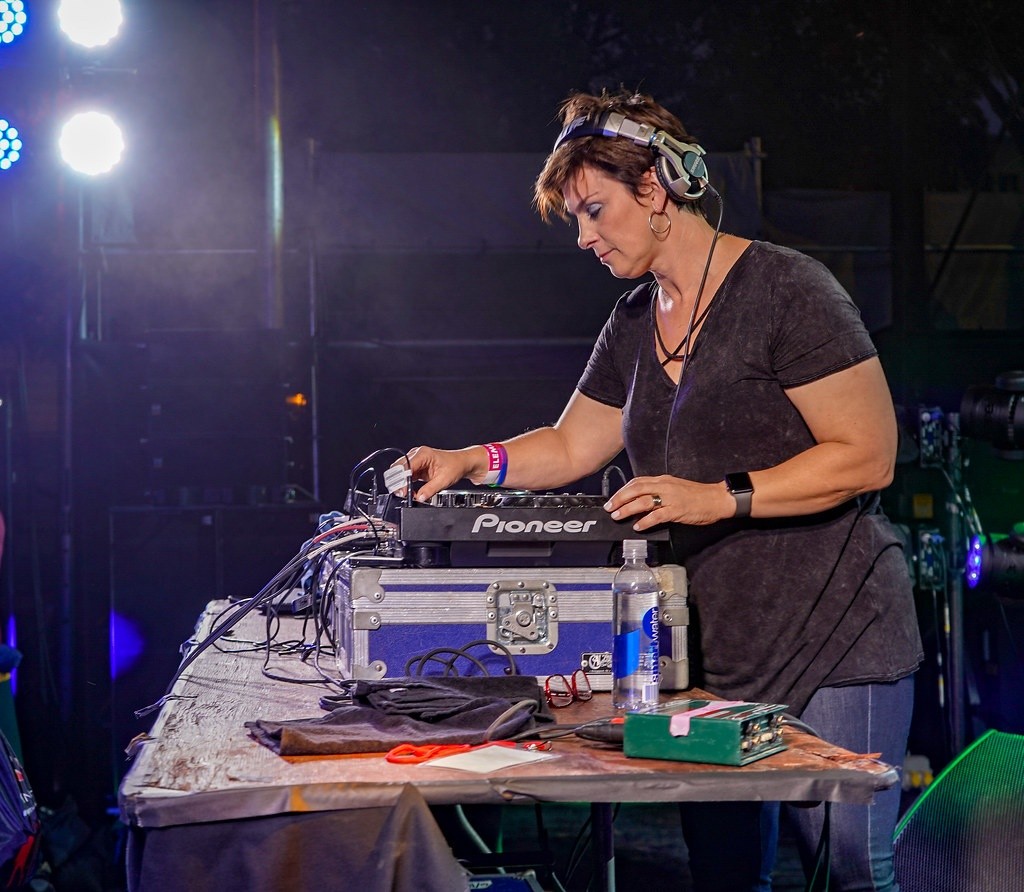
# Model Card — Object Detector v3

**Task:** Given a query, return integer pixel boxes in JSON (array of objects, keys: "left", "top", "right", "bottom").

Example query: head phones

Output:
[{"left": 553, "top": 111, "right": 708, "bottom": 205}]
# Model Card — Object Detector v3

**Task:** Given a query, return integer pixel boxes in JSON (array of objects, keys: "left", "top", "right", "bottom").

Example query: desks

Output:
[{"left": 116, "top": 598, "right": 899, "bottom": 892}]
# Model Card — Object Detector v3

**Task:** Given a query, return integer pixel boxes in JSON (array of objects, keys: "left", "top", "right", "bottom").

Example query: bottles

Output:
[{"left": 613, "top": 539, "right": 659, "bottom": 709}]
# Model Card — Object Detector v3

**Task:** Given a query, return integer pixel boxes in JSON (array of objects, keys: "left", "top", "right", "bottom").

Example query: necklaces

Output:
[{"left": 655, "top": 284, "right": 721, "bottom": 367}]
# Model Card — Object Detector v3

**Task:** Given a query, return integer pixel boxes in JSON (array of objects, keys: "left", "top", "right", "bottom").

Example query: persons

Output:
[{"left": 382, "top": 93, "right": 923, "bottom": 892}]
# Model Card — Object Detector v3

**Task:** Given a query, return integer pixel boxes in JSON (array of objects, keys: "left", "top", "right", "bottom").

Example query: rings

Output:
[{"left": 652, "top": 495, "right": 662, "bottom": 509}]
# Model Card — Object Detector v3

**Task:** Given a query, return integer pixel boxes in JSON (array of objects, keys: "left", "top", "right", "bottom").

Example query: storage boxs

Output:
[{"left": 312, "top": 544, "right": 691, "bottom": 699}]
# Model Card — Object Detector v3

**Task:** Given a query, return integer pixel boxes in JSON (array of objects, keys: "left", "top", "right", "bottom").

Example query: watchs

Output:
[{"left": 724, "top": 471, "right": 755, "bottom": 518}]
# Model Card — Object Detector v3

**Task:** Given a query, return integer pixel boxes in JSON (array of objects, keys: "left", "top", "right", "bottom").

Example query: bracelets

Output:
[{"left": 470, "top": 443, "right": 508, "bottom": 487}]
